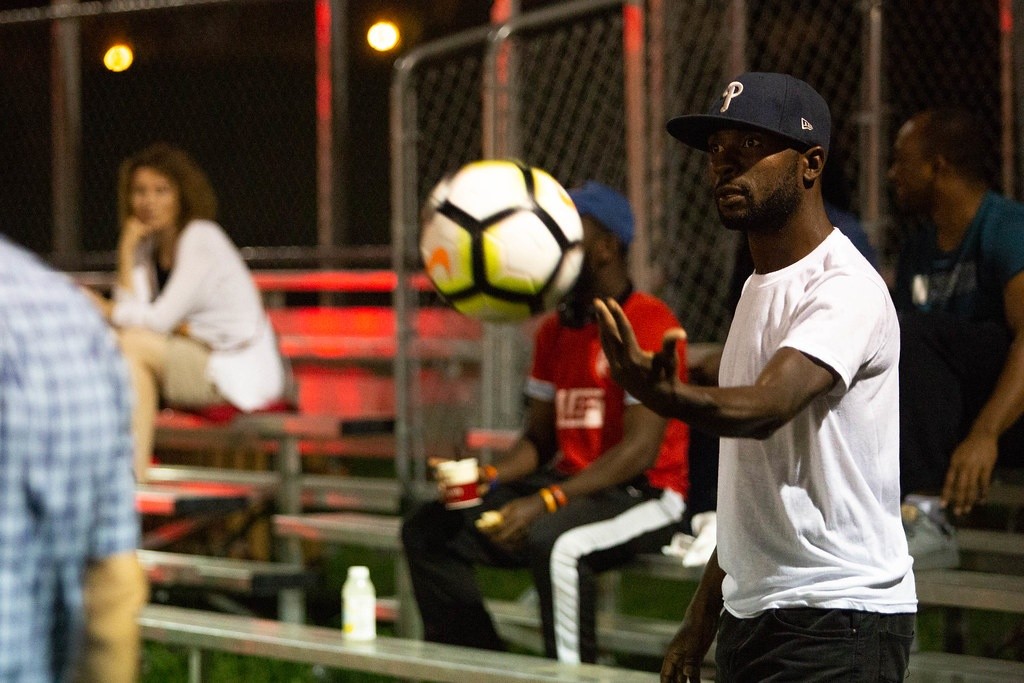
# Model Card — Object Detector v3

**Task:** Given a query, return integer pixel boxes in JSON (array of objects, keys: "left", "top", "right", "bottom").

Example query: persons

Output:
[
  {"left": 0, "top": 223, "right": 147, "bottom": 683},
  {"left": 80, "top": 146, "right": 284, "bottom": 484},
  {"left": 592, "top": 74, "right": 918, "bottom": 683},
  {"left": 877, "top": 99, "right": 1024, "bottom": 523},
  {"left": 399, "top": 183, "right": 691, "bottom": 665}
]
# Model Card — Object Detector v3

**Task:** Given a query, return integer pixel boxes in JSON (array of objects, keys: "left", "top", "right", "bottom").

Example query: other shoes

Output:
[{"left": 902, "top": 494, "right": 961, "bottom": 570}]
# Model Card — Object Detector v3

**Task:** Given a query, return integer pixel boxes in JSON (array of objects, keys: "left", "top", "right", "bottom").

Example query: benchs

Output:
[{"left": 138, "top": 268, "right": 1024, "bottom": 683}]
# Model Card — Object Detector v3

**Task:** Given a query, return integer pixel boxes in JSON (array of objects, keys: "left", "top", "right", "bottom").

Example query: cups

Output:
[{"left": 435, "top": 455, "right": 484, "bottom": 511}]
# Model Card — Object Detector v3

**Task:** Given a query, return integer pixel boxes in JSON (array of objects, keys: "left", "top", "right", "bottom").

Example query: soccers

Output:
[{"left": 419, "top": 157, "right": 587, "bottom": 329}]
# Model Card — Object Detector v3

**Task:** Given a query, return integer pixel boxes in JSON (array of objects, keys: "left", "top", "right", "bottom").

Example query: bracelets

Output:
[{"left": 540, "top": 484, "right": 567, "bottom": 513}]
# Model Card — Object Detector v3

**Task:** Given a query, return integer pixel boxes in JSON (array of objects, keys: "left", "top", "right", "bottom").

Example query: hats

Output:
[
  {"left": 667, "top": 71, "right": 831, "bottom": 162},
  {"left": 563, "top": 180, "right": 634, "bottom": 246}
]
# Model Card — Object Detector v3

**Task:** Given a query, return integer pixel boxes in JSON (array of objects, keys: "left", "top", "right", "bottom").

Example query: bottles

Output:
[{"left": 340, "top": 564, "right": 377, "bottom": 643}]
